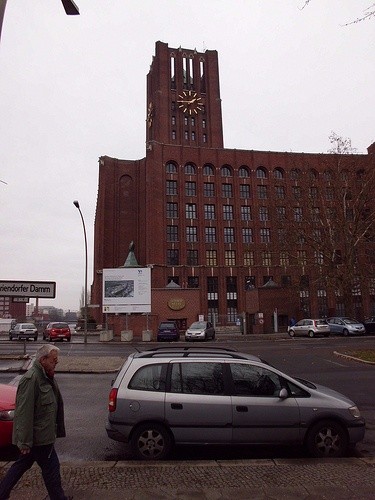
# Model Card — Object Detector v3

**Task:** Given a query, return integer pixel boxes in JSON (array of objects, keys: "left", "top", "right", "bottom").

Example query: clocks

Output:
[
  {"left": 146, "top": 101, "right": 155, "bottom": 131},
  {"left": 175, "top": 90, "right": 205, "bottom": 118}
]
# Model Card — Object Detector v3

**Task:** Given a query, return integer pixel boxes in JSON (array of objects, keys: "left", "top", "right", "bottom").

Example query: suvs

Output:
[
  {"left": 105, "top": 345, "right": 366, "bottom": 458},
  {"left": 157, "top": 321, "right": 181, "bottom": 342}
]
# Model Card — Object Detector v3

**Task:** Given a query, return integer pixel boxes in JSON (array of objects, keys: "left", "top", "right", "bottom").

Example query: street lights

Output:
[{"left": 73, "top": 200, "right": 88, "bottom": 344}]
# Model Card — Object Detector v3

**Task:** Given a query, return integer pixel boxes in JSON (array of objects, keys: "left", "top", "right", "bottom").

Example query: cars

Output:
[
  {"left": 362, "top": 316, "right": 375, "bottom": 333},
  {"left": 185, "top": 321, "right": 216, "bottom": 342},
  {"left": 42, "top": 322, "right": 72, "bottom": 342},
  {"left": 325, "top": 316, "right": 366, "bottom": 337},
  {"left": 288, "top": 318, "right": 331, "bottom": 338},
  {"left": 0, "top": 383, "right": 17, "bottom": 453},
  {"left": 8, "top": 323, "right": 38, "bottom": 342}
]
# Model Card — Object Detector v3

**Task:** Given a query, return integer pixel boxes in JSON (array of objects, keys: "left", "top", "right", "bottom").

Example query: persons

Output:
[
  {"left": 289, "top": 318, "right": 295, "bottom": 326},
  {"left": 0, "top": 344, "right": 66, "bottom": 500}
]
